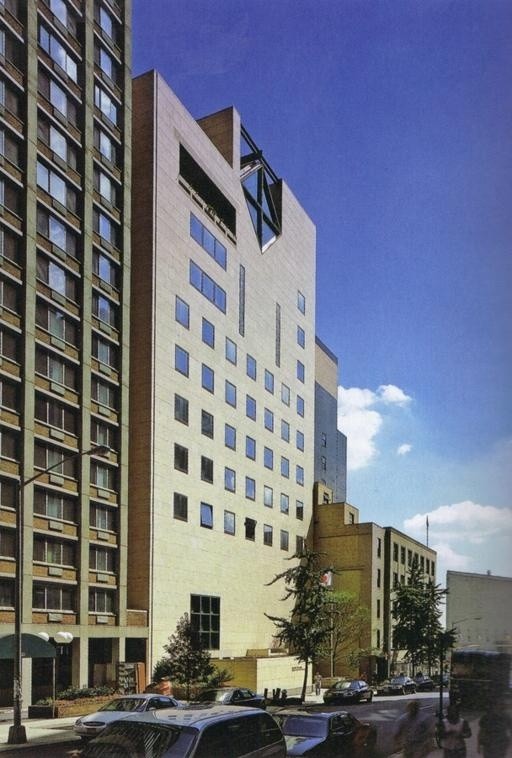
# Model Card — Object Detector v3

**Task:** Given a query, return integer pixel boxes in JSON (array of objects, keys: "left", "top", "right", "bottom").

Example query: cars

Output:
[
  {"left": 376, "top": 674, "right": 449, "bottom": 695},
  {"left": 323, "top": 680, "right": 373, "bottom": 706}
]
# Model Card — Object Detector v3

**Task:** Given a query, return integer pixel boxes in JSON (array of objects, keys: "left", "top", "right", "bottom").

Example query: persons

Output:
[
  {"left": 417, "top": 670, "right": 422, "bottom": 676},
  {"left": 314, "top": 672, "right": 322, "bottom": 695},
  {"left": 476, "top": 698, "right": 512, "bottom": 758},
  {"left": 397, "top": 672, "right": 400, "bottom": 677},
  {"left": 358, "top": 670, "right": 386, "bottom": 684},
  {"left": 391, "top": 672, "right": 395, "bottom": 677},
  {"left": 392, "top": 701, "right": 434, "bottom": 758},
  {"left": 439, "top": 705, "right": 472, "bottom": 758}
]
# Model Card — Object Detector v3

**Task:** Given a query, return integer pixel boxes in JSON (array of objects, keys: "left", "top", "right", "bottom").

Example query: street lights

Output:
[
  {"left": 36, "top": 631, "right": 74, "bottom": 718},
  {"left": 8, "top": 444, "right": 111, "bottom": 744}
]
